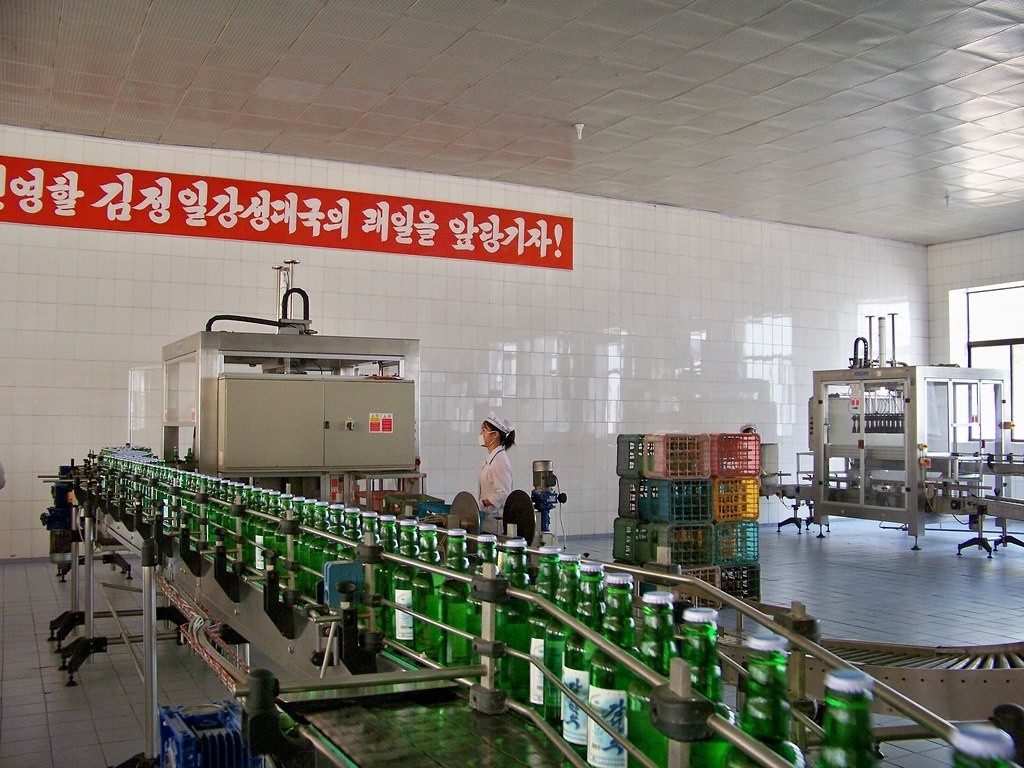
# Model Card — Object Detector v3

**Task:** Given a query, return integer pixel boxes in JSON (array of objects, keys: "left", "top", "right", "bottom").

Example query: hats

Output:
[{"left": 485, "top": 410, "right": 515, "bottom": 434}]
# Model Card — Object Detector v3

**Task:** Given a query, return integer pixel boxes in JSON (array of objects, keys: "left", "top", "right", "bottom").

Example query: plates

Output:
[{"left": 92, "top": 432, "right": 1015, "bottom": 768}]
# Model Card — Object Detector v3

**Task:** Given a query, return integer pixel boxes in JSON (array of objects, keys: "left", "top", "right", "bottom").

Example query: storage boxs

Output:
[
  {"left": 354, "top": 489, "right": 486, "bottom": 537},
  {"left": 612, "top": 431, "right": 761, "bottom": 612}
]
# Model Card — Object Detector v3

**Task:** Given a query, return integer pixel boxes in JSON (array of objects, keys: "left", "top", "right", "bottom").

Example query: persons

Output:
[{"left": 478, "top": 417, "right": 516, "bottom": 534}]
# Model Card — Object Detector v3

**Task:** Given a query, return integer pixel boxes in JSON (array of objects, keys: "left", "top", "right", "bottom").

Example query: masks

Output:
[{"left": 478, "top": 431, "right": 496, "bottom": 446}]
